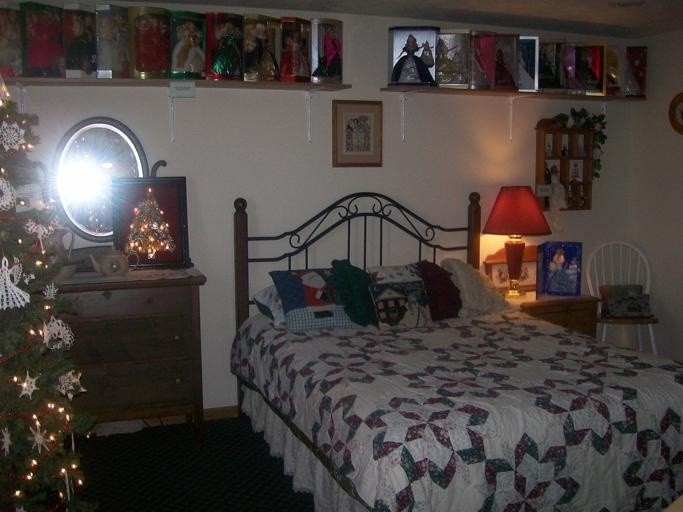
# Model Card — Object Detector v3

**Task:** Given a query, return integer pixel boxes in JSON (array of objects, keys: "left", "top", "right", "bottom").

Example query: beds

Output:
[{"left": 228, "top": 189, "right": 683, "bottom": 512}]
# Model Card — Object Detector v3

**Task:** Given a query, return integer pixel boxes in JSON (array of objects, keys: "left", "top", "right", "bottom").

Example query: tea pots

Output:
[{"left": 89, "top": 249, "right": 139, "bottom": 276}]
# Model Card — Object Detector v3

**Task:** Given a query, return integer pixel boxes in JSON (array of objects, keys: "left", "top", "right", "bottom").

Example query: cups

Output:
[{"left": 60, "top": 265, "right": 74, "bottom": 280}]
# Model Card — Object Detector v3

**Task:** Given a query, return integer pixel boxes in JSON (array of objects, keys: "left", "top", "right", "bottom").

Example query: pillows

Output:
[
  {"left": 596, "top": 284, "right": 645, "bottom": 321},
  {"left": 251, "top": 256, "right": 523, "bottom": 337}
]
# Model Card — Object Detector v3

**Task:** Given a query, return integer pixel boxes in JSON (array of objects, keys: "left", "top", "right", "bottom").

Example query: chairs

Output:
[{"left": 582, "top": 240, "right": 660, "bottom": 355}]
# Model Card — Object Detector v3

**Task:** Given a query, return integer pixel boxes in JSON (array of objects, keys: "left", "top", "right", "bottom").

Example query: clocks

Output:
[{"left": 667, "top": 92, "right": 683, "bottom": 136}]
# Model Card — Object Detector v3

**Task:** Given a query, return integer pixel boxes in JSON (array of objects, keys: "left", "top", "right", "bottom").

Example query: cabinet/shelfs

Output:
[
  {"left": 533, "top": 124, "right": 596, "bottom": 213},
  {"left": 22, "top": 268, "right": 209, "bottom": 430}
]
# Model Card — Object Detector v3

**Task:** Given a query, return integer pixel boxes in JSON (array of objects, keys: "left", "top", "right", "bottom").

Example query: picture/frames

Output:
[
  {"left": 111, "top": 176, "right": 191, "bottom": 271},
  {"left": 330, "top": 97, "right": 384, "bottom": 169}
]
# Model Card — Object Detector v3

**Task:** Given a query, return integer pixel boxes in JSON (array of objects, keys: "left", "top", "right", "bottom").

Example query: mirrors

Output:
[{"left": 50, "top": 113, "right": 152, "bottom": 245}]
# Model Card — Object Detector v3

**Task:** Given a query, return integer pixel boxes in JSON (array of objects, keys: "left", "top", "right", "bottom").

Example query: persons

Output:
[
  {"left": 2, "top": 10, "right": 342, "bottom": 77},
  {"left": 390, "top": 33, "right": 622, "bottom": 98},
  {"left": 546, "top": 247, "right": 577, "bottom": 294},
  {"left": 2, "top": 2, "right": 342, "bottom": 85},
  {"left": 543, "top": 160, "right": 584, "bottom": 233}
]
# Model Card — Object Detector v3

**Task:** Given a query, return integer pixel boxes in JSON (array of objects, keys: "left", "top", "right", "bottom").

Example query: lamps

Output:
[{"left": 481, "top": 184, "right": 553, "bottom": 299}]
